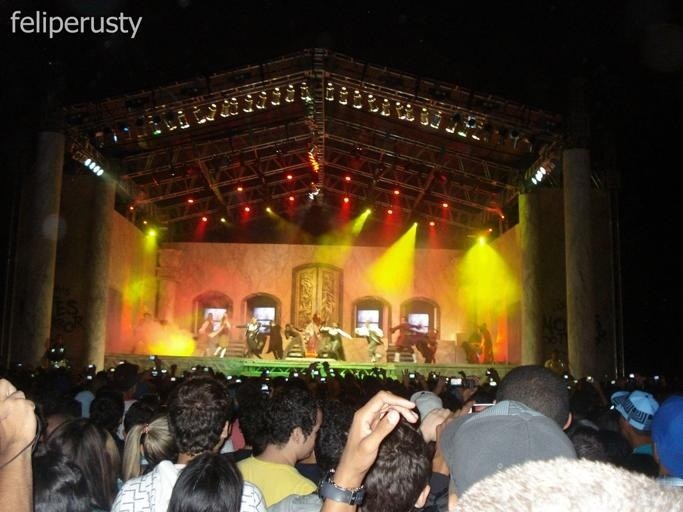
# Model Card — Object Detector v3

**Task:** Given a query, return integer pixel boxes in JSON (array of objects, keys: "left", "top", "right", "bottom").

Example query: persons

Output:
[
  {"left": 610, "top": 389, "right": 658, "bottom": 478},
  {"left": 304, "top": 313, "right": 325, "bottom": 355},
  {"left": 209, "top": 312, "right": 232, "bottom": 358},
  {"left": 389, "top": 313, "right": 422, "bottom": 364},
  {"left": 312, "top": 405, "right": 359, "bottom": 476},
  {"left": 545, "top": 346, "right": 565, "bottom": 376},
  {"left": 428, "top": 365, "right": 500, "bottom": 414},
  {"left": 577, "top": 366, "right": 682, "bottom": 407},
  {"left": 261, "top": 320, "right": 284, "bottom": 360},
  {"left": 356, "top": 419, "right": 432, "bottom": 511},
  {"left": 283, "top": 324, "right": 307, "bottom": 359},
  {"left": 452, "top": 456, "right": 683, "bottom": 512},
  {"left": 320, "top": 362, "right": 444, "bottom": 421},
  {"left": 130, "top": 313, "right": 153, "bottom": 353},
  {"left": 418, "top": 407, "right": 453, "bottom": 446},
  {"left": 3, "top": 355, "right": 322, "bottom": 512},
  {"left": 354, "top": 320, "right": 385, "bottom": 364},
  {"left": 565, "top": 395, "right": 614, "bottom": 465},
  {"left": 235, "top": 317, "right": 264, "bottom": 362},
  {"left": 421, "top": 364, "right": 574, "bottom": 511},
  {"left": 194, "top": 312, "right": 215, "bottom": 355},
  {"left": 461, "top": 320, "right": 495, "bottom": 364},
  {"left": 417, "top": 326, "right": 439, "bottom": 365},
  {"left": 320, "top": 390, "right": 581, "bottom": 512},
  {"left": 319, "top": 322, "right": 352, "bottom": 361},
  {"left": 586, "top": 376, "right": 635, "bottom": 470}
]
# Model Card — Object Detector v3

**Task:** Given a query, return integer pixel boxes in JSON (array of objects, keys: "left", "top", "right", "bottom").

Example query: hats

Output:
[
  {"left": 409, "top": 390, "right": 444, "bottom": 422},
  {"left": 437, "top": 399, "right": 580, "bottom": 499},
  {"left": 609, "top": 389, "right": 659, "bottom": 433}
]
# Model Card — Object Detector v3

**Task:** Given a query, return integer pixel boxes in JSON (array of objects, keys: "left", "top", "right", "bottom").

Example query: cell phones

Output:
[
  {"left": 468, "top": 403, "right": 497, "bottom": 414},
  {"left": 17, "top": 355, "right": 660, "bottom": 390}
]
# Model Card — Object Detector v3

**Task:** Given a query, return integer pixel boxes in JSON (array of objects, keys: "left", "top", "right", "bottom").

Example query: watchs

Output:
[{"left": 316, "top": 468, "right": 365, "bottom": 507}]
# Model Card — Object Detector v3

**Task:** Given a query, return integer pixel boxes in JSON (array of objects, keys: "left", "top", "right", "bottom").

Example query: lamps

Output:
[
  {"left": 162, "top": 113, "right": 177, "bottom": 132},
  {"left": 353, "top": 91, "right": 362, "bottom": 109},
  {"left": 148, "top": 116, "right": 164, "bottom": 137},
  {"left": 395, "top": 102, "right": 406, "bottom": 119},
  {"left": 256, "top": 91, "right": 266, "bottom": 109},
  {"left": 135, "top": 117, "right": 148, "bottom": 140},
  {"left": 229, "top": 98, "right": 239, "bottom": 116},
  {"left": 446, "top": 112, "right": 461, "bottom": 133},
  {"left": 301, "top": 83, "right": 309, "bottom": 100},
  {"left": 472, "top": 122, "right": 484, "bottom": 141},
  {"left": 326, "top": 83, "right": 334, "bottom": 101},
  {"left": 368, "top": 95, "right": 379, "bottom": 112},
  {"left": 430, "top": 111, "right": 443, "bottom": 129},
  {"left": 206, "top": 103, "right": 217, "bottom": 122},
  {"left": 405, "top": 104, "right": 415, "bottom": 122},
  {"left": 243, "top": 95, "right": 253, "bottom": 113},
  {"left": 381, "top": 99, "right": 390, "bottom": 116},
  {"left": 339, "top": 87, "right": 348, "bottom": 105},
  {"left": 219, "top": 100, "right": 231, "bottom": 117},
  {"left": 192, "top": 107, "right": 206, "bottom": 125},
  {"left": 178, "top": 109, "right": 190, "bottom": 130},
  {"left": 529, "top": 161, "right": 555, "bottom": 186},
  {"left": 458, "top": 119, "right": 470, "bottom": 137},
  {"left": 420, "top": 108, "right": 429, "bottom": 125},
  {"left": 72, "top": 151, "right": 105, "bottom": 176},
  {"left": 285, "top": 85, "right": 296, "bottom": 103},
  {"left": 271, "top": 87, "right": 282, "bottom": 106},
  {"left": 122, "top": 121, "right": 135, "bottom": 140}
]
